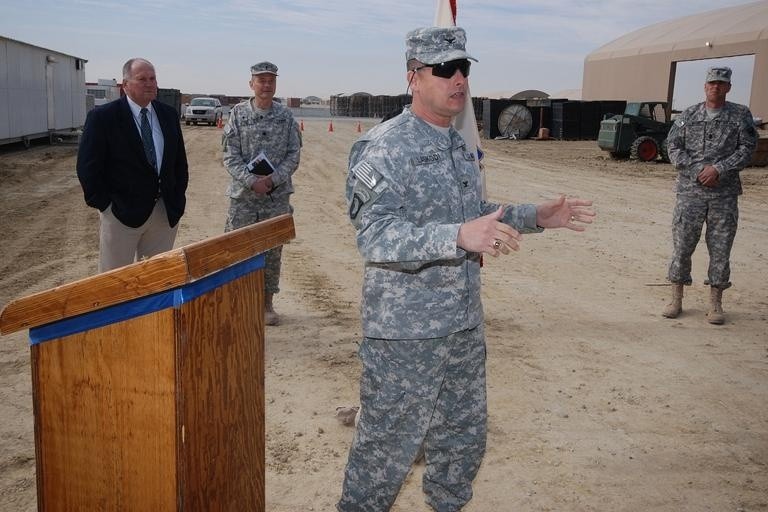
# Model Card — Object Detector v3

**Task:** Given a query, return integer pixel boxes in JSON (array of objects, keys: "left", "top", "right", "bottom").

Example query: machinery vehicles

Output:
[{"left": 597, "top": 99, "right": 677, "bottom": 164}]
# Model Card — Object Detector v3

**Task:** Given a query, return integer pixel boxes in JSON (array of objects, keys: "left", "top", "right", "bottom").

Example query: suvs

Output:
[{"left": 183, "top": 96, "right": 225, "bottom": 126}]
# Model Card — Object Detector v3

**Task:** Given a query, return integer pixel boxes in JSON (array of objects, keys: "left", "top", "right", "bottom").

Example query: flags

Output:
[{"left": 430, "top": 0, "right": 486, "bottom": 269}]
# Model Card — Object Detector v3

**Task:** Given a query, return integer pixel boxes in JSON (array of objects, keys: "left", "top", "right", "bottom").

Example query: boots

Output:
[
  {"left": 705, "top": 285, "right": 727, "bottom": 324},
  {"left": 662, "top": 279, "right": 683, "bottom": 319},
  {"left": 265, "top": 292, "right": 279, "bottom": 326}
]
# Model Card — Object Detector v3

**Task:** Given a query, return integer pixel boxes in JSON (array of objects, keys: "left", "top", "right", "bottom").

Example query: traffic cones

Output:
[
  {"left": 328, "top": 119, "right": 335, "bottom": 132},
  {"left": 216, "top": 114, "right": 224, "bottom": 128},
  {"left": 356, "top": 120, "right": 362, "bottom": 132},
  {"left": 300, "top": 119, "right": 305, "bottom": 131}
]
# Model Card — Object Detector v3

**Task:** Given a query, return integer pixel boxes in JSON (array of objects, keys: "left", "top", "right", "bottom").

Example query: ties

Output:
[{"left": 140, "top": 108, "right": 157, "bottom": 175}]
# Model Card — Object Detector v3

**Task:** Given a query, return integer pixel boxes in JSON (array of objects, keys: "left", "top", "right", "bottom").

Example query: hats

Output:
[
  {"left": 405, "top": 25, "right": 480, "bottom": 65},
  {"left": 706, "top": 65, "right": 733, "bottom": 85},
  {"left": 251, "top": 60, "right": 280, "bottom": 76}
]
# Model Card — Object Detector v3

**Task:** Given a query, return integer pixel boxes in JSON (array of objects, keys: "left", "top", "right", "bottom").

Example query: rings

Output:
[
  {"left": 570, "top": 214, "right": 576, "bottom": 222},
  {"left": 493, "top": 237, "right": 501, "bottom": 250}
]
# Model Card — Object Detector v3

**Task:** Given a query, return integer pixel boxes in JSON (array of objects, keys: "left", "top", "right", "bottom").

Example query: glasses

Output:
[{"left": 413, "top": 60, "right": 471, "bottom": 79}]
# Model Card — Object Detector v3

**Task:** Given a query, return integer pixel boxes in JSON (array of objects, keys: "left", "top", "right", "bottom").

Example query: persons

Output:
[
  {"left": 661, "top": 66, "right": 758, "bottom": 326},
  {"left": 77, "top": 58, "right": 189, "bottom": 272},
  {"left": 337, "top": 26, "right": 597, "bottom": 512},
  {"left": 220, "top": 62, "right": 303, "bottom": 326}
]
